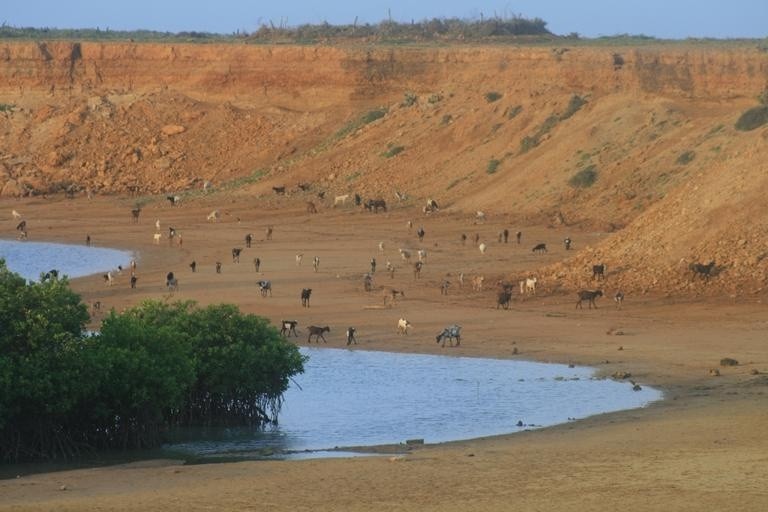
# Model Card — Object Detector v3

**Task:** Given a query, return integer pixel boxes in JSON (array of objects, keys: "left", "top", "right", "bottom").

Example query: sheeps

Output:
[
  {"left": 311, "top": 255, "right": 321, "bottom": 273},
  {"left": 231, "top": 247, "right": 243, "bottom": 265},
  {"left": 436, "top": 324, "right": 463, "bottom": 348},
  {"left": 590, "top": 264, "right": 605, "bottom": 282},
  {"left": 255, "top": 280, "right": 273, "bottom": 298},
  {"left": 254, "top": 257, "right": 261, "bottom": 272},
  {"left": 396, "top": 318, "right": 412, "bottom": 337},
  {"left": 265, "top": 224, "right": 274, "bottom": 241},
  {"left": 300, "top": 288, "right": 313, "bottom": 308},
  {"left": 40, "top": 268, "right": 59, "bottom": 285},
  {"left": 345, "top": 326, "right": 357, "bottom": 347},
  {"left": 613, "top": 291, "right": 623, "bottom": 307},
  {"left": 688, "top": 256, "right": 717, "bottom": 281},
  {"left": 305, "top": 326, "right": 332, "bottom": 343},
  {"left": 295, "top": 253, "right": 305, "bottom": 270},
  {"left": 188, "top": 261, "right": 196, "bottom": 273},
  {"left": 83, "top": 181, "right": 185, "bottom": 317},
  {"left": 215, "top": 261, "right": 222, "bottom": 274},
  {"left": 563, "top": 237, "right": 572, "bottom": 250},
  {"left": 362, "top": 210, "right": 548, "bottom": 307},
  {"left": 574, "top": 290, "right": 604, "bottom": 310},
  {"left": 270, "top": 181, "right": 441, "bottom": 215},
  {"left": 245, "top": 233, "right": 255, "bottom": 248},
  {"left": 10, "top": 208, "right": 29, "bottom": 242},
  {"left": 279, "top": 320, "right": 300, "bottom": 339}
]
